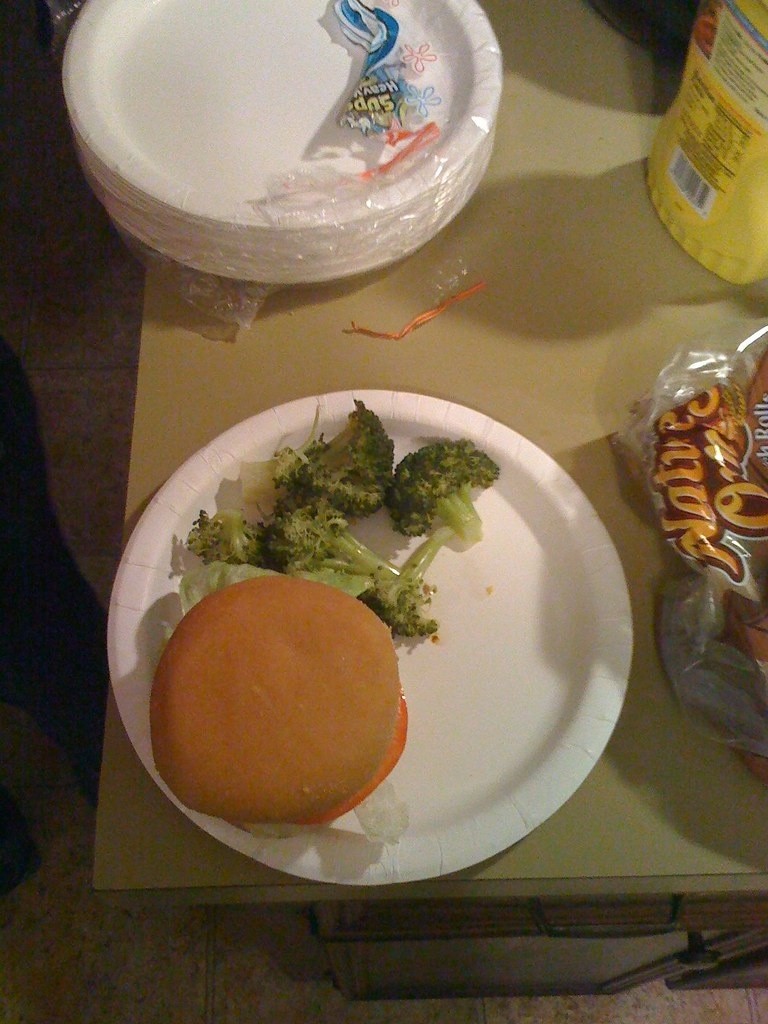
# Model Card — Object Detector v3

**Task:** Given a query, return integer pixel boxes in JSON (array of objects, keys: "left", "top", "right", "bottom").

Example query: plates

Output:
[
  {"left": 107, "top": 390, "right": 634, "bottom": 884},
  {"left": 62, "top": 0, "right": 504, "bottom": 284}
]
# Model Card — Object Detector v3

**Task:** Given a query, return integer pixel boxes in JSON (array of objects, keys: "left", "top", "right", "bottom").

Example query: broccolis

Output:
[{"left": 184, "top": 410, "right": 500, "bottom": 630}]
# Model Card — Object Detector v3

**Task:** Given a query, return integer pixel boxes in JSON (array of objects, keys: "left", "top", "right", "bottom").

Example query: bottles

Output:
[{"left": 647, "top": 2, "right": 768, "bottom": 294}]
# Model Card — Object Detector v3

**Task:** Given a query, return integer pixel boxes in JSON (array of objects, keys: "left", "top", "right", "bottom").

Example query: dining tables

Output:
[{"left": 90, "top": 0, "right": 768, "bottom": 1000}]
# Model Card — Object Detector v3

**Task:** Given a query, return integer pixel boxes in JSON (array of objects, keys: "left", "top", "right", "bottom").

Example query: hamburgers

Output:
[{"left": 152, "top": 562, "right": 406, "bottom": 828}]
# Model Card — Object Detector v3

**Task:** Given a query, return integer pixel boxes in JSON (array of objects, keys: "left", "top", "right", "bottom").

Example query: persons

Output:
[{"left": 0, "top": 337, "right": 110, "bottom": 896}]
